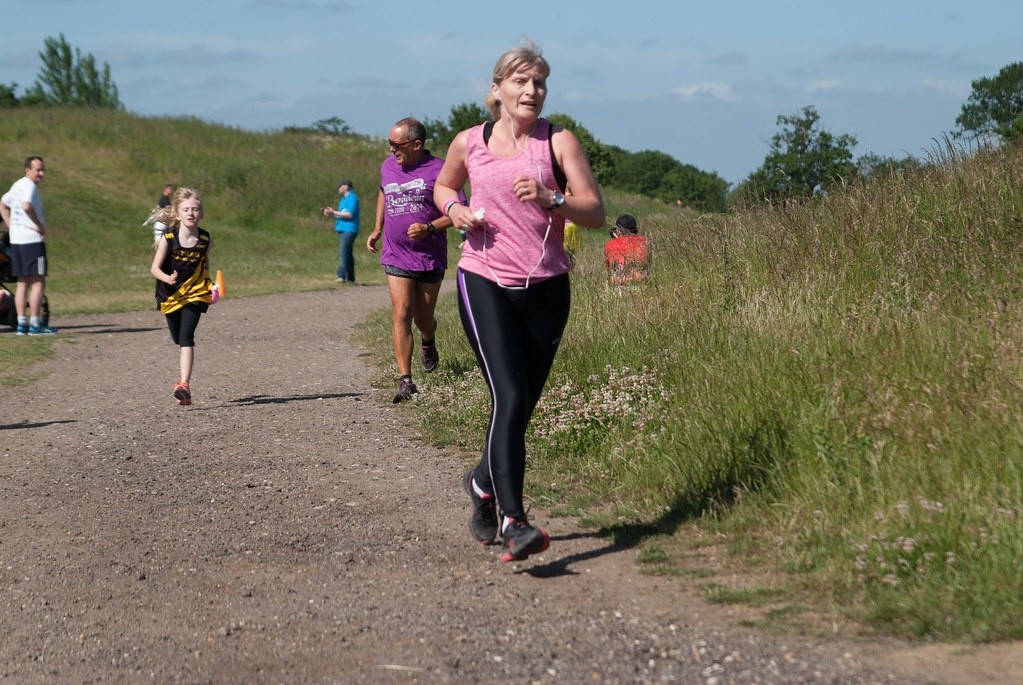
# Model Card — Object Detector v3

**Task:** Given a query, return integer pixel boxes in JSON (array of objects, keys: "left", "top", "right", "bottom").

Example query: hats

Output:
[
  {"left": 338, "top": 179, "right": 355, "bottom": 188},
  {"left": 616, "top": 214, "right": 638, "bottom": 234}
]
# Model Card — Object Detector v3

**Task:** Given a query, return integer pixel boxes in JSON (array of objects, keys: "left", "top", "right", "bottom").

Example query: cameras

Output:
[{"left": 610, "top": 226, "right": 617, "bottom": 238}]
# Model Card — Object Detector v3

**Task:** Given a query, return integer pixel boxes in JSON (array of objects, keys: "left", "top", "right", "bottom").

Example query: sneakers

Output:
[
  {"left": 15, "top": 322, "right": 58, "bottom": 336},
  {"left": 421, "top": 338, "right": 439, "bottom": 373},
  {"left": 174, "top": 383, "right": 193, "bottom": 405},
  {"left": 501, "top": 518, "right": 551, "bottom": 564},
  {"left": 392, "top": 382, "right": 417, "bottom": 405},
  {"left": 463, "top": 468, "right": 499, "bottom": 545}
]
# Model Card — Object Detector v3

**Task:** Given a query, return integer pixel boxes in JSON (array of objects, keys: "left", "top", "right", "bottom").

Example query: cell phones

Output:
[{"left": 461, "top": 206, "right": 486, "bottom": 234}]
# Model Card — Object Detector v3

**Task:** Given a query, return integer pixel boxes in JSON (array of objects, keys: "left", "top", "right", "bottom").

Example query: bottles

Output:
[{"left": 211, "top": 283, "right": 219, "bottom": 303}]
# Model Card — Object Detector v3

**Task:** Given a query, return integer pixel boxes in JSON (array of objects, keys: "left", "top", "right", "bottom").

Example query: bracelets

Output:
[{"left": 443, "top": 199, "right": 460, "bottom": 217}]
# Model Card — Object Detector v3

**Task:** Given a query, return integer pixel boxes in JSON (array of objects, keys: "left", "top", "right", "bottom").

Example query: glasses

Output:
[{"left": 388, "top": 137, "right": 419, "bottom": 148}]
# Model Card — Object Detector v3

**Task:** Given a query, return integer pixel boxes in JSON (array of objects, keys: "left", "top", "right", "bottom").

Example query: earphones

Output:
[{"left": 495, "top": 90, "right": 499, "bottom": 95}]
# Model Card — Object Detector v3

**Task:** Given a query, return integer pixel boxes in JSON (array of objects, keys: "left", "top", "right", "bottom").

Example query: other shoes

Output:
[{"left": 335, "top": 277, "right": 355, "bottom": 283}]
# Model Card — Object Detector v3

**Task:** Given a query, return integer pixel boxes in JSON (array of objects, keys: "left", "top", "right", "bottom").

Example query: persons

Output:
[
  {"left": 433, "top": 46, "right": 606, "bottom": 564},
  {"left": 0, "top": 156, "right": 59, "bottom": 337},
  {"left": 368, "top": 118, "right": 468, "bottom": 404},
  {"left": 141, "top": 184, "right": 213, "bottom": 405},
  {"left": 602, "top": 215, "right": 652, "bottom": 288},
  {"left": 323, "top": 180, "right": 360, "bottom": 283}
]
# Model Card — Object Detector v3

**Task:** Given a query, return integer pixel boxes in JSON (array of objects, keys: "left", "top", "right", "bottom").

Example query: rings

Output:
[
  {"left": 463, "top": 224, "right": 468, "bottom": 229},
  {"left": 524, "top": 187, "right": 527, "bottom": 194}
]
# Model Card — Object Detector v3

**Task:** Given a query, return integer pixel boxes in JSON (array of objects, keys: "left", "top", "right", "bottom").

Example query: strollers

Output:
[{"left": 0, "top": 231, "right": 51, "bottom": 331}]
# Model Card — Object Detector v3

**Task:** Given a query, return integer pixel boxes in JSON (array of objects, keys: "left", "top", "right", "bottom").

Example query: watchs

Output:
[
  {"left": 427, "top": 223, "right": 436, "bottom": 235},
  {"left": 547, "top": 189, "right": 565, "bottom": 212}
]
